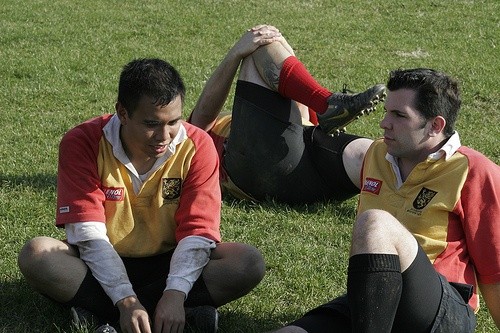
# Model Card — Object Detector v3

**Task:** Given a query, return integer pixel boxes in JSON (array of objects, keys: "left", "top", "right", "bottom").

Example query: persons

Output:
[
  {"left": 18, "top": 59, "right": 264, "bottom": 333},
  {"left": 275, "top": 68, "right": 500, "bottom": 333},
  {"left": 188, "top": 25, "right": 386, "bottom": 207}
]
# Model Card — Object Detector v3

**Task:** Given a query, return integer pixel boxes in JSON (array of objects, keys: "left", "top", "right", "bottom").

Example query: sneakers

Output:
[
  {"left": 316, "top": 83, "right": 386, "bottom": 134},
  {"left": 182, "top": 307, "right": 218, "bottom": 333},
  {"left": 96, "top": 324, "right": 117, "bottom": 333}
]
